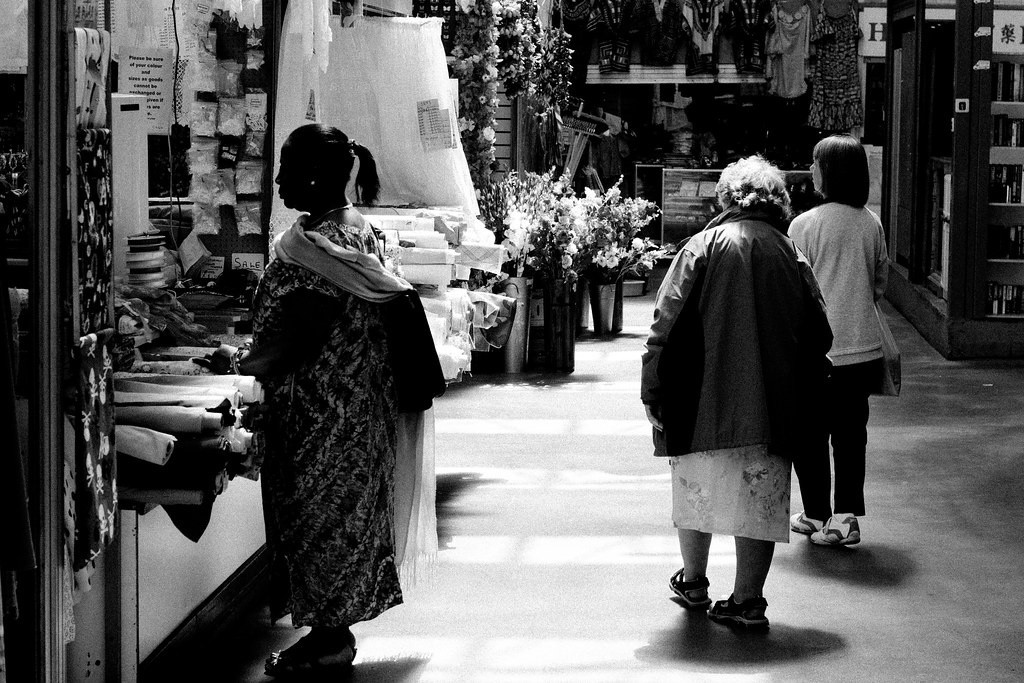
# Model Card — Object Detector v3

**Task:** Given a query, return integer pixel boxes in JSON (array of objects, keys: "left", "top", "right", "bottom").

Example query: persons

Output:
[
  {"left": 235, "top": 124, "right": 439, "bottom": 673},
  {"left": 787, "top": 136, "right": 889, "bottom": 545},
  {"left": 640, "top": 155, "right": 835, "bottom": 624}
]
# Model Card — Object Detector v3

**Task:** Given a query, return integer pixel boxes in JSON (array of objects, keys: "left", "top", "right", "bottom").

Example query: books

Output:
[{"left": 981, "top": 60, "right": 1023, "bottom": 316}]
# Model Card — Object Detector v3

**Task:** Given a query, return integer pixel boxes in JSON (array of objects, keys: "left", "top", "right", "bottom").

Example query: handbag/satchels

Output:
[{"left": 868, "top": 300, "right": 901, "bottom": 396}]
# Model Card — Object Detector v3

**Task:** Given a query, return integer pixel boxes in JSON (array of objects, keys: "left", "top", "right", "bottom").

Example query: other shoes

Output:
[{"left": 265, "top": 634, "right": 356, "bottom": 675}]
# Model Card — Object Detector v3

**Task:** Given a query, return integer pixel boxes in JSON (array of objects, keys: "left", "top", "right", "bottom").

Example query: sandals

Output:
[
  {"left": 710, "top": 593, "right": 769, "bottom": 623},
  {"left": 668, "top": 566, "right": 713, "bottom": 607}
]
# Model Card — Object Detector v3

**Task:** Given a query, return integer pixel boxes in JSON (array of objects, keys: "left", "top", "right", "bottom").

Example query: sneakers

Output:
[
  {"left": 810, "top": 515, "right": 860, "bottom": 546},
  {"left": 790, "top": 510, "right": 827, "bottom": 533}
]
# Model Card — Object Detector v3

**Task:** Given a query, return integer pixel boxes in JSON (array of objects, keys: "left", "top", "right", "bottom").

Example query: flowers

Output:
[
  {"left": 474, "top": 164, "right": 678, "bottom": 293},
  {"left": 436, "top": 1, "right": 572, "bottom": 185}
]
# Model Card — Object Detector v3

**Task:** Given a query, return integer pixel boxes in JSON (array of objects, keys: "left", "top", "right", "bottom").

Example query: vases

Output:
[{"left": 525, "top": 279, "right": 622, "bottom": 374}]
